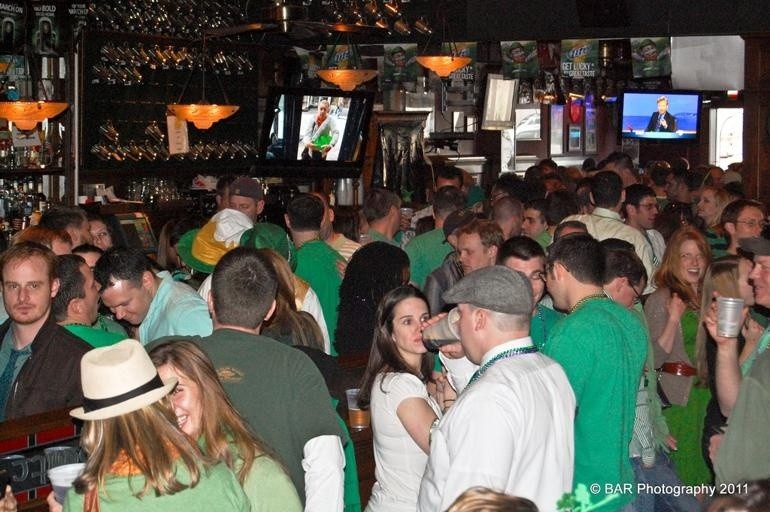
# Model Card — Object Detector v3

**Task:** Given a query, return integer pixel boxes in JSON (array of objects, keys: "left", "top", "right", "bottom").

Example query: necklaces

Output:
[
  {"left": 571, "top": 294, "right": 608, "bottom": 314},
  {"left": 461, "top": 344, "right": 541, "bottom": 390}
]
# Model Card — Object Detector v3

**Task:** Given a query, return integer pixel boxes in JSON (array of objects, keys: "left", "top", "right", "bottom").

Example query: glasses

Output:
[
  {"left": 617, "top": 274, "right": 642, "bottom": 304},
  {"left": 632, "top": 203, "right": 660, "bottom": 209},
  {"left": 539, "top": 263, "right": 569, "bottom": 282},
  {"left": 737, "top": 219, "right": 767, "bottom": 227},
  {"left": 489, "top": 192, "right": 506, "bottom": 199}
]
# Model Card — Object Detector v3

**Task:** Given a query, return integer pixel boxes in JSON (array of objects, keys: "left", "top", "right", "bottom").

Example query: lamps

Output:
[
  {"left": 1, "top": 2, "right": 71, "bottom": 131},
  {"left": 166, "top": 32, "right": 240, "bottom": 130},
  {"left": 316, "top": 31, "right": 378, "bottom": 92},
  {"left": 415, "top": 4, "right": 472, "bottom": 77}
]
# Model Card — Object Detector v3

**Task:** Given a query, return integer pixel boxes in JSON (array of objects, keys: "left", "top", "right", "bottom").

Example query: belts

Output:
[{"left": 661, "top": 362, "right": 695, "bottom": 376}]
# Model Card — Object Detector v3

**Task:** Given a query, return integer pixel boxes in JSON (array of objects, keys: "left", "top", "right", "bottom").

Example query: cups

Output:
[
  {"left": 422, "top": 307, "right": 462, "bottom": 353},
  {"left": 715, "top": 296, "right": 743, "bottom": 339},
  {"left": 401, "top": 207, "right": 413, "bottom": 225},
  {"left": 345, "top": 388, "right": 372, "bottom": 428},
  {"left": 43, "top": 445, "right": 87, "bottom": 505}
]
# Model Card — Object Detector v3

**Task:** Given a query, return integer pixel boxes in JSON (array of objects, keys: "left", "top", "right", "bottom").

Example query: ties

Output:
[{"left": 0, "top": 349, "right": 20, "bottom": 420}]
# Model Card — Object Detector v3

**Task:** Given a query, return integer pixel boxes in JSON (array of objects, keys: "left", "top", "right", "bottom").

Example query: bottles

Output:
[
  {"left": 87, "top": 119, "right": 261, "bottom": 163},
  {"left": 0, "top": 177, "right": 46, "bottom": 235},
  {"left": 1, "top": 145, "right": 42, "bottom": 171},
  {"left": 88, "top": 44, "right": 254, "bottom": 86},
  {"left": 326, "top": 1, "right": 435, "bottom": 38},
  {"left": 119, "top": 177, "right": 179, "bottom": 208},
  {"left": 86, "top": 1, "right": 249, "bottom": 41}
]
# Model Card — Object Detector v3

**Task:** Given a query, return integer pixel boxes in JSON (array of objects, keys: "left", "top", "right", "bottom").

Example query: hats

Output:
[
  {"left": 442, "top": 265, "right": 535, "bottom": 313},
  {"left": 229, "top": 178, "right": 264, "bottom": 199},
  {"left": 443, "top": 209, "right": 478, "bottom": 238},
  {"left": 177, "top": 209, "right": 253, "bottom": 274},
  {"left": 240, "top": 223, "right": 298, "bottom": 273},
  {"left": 738, "top": 225, "right": 770, "bottom": 256},
  {"left": 69, "top": 338, "right": 178, "bottom": 421}
]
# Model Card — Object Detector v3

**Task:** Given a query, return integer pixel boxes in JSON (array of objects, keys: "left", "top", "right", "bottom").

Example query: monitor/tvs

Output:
[
  {"left": 617, "top": 88, "right": 704, "bottom": 145},
  {"left": 259, "top": 86, "right": 376, "bottom": 179}
]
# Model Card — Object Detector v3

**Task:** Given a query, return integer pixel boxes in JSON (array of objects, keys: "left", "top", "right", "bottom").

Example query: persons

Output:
[
  {"left": 713, "top": 199, "right": 764, "bottom": 259},
  {"left": 39, "top": 205, "right": 92, "bottom": 250},
  {"left": 302, "top": 100, "right": 338, "bottom": 160},
  {"left": 643, "top": 226, "right": 710, "bottom": 504},
  {"left": 408, "top": 187, "right": 469, "bottom": 290},
  {"left": 494, "top": 237, "right": 566, "bottom": 350},
  {"left": 334, "top": 241, "right": 411, "bottom": 389},
  {"left": 229, "top": 177, "right": 265, "bottom": 223},
  {"left": 363, "top": 189, "right": 410, "bottom": 252},
  {"left": 354, "top": 286, "right": 444, "bottom": 511},
  {"left": 47, "top": 338, "right": 251, "bottom": 512},
  {"left": 148, "top": 341, "right": 303, "bottom": 512},
  {"left": 310, "top": 191, "right": 361, "bottom": 281},
  {"left": 258, "top": 248, "right": 325, "bottom": 355},
  {"left": 196, "top": 223, "right": 331, "bottom": 356},
  {"left": 53, "top": 254, "right": 129, "bottom": 347},
  {"left": 0, "top": 240, "right": 96, "bottom": 423},
  {"left": 701, "top": 255, "right": 765, "bottom": 490},
  {"left": 644, "top": 96, "right": 677, "bottom": 133},
  {"left": 703, "top": 224, "right": 770, "bottom": 512},
  {"left": 545, "top": 234, "right": 649, "bottom": 511},
  {"left": 94, "top": 246, "right": 213, "bottom": 346},
  {"left": 284, "top": 192, "right": 350, "bottom": 357},
  {"left": 87, "top": 214, "right": 129, "bottom": 251},
  {"left": 12, "top": 225, "right": 73, "bottom": 256},
  {"left": 458, "top": 220, "right": 504, "bottom": 276},
  {"left": 215, "top": 176, "right": 238, "bottom": 212},
  {"left": 540, "top": 250, "right": 648, "bottom": 310},
  {"left": 134, "top": 207, "right": 254, "bottom": 344},
  {"left": 72, "top": 243, "right": 104, "bottom": 274},
  {"left": 157, "top": 219, "right": 178, "bottom": 269},
  {"left": 600, "top": 239, "right": 704, "bottom": 511},
  {"left": 145, "top": 248, "right": 348, "bottom": 511},
  {"left": 170, "top": 220, "right": 207, "bottom": 273},
  {"left": 424, "top": 211, "right": 478, "bottom": 317},
  {"left": 437, "top": 153, "right": 765, "bottom": 276}
]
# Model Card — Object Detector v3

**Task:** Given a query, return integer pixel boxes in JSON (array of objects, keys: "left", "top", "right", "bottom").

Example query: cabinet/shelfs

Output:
[
  {"left": 77, "top": 26, "right": 259, "bottom": 213},
  {"left": 0, "top": 54, "right": 74, "bottom": 249}
]
